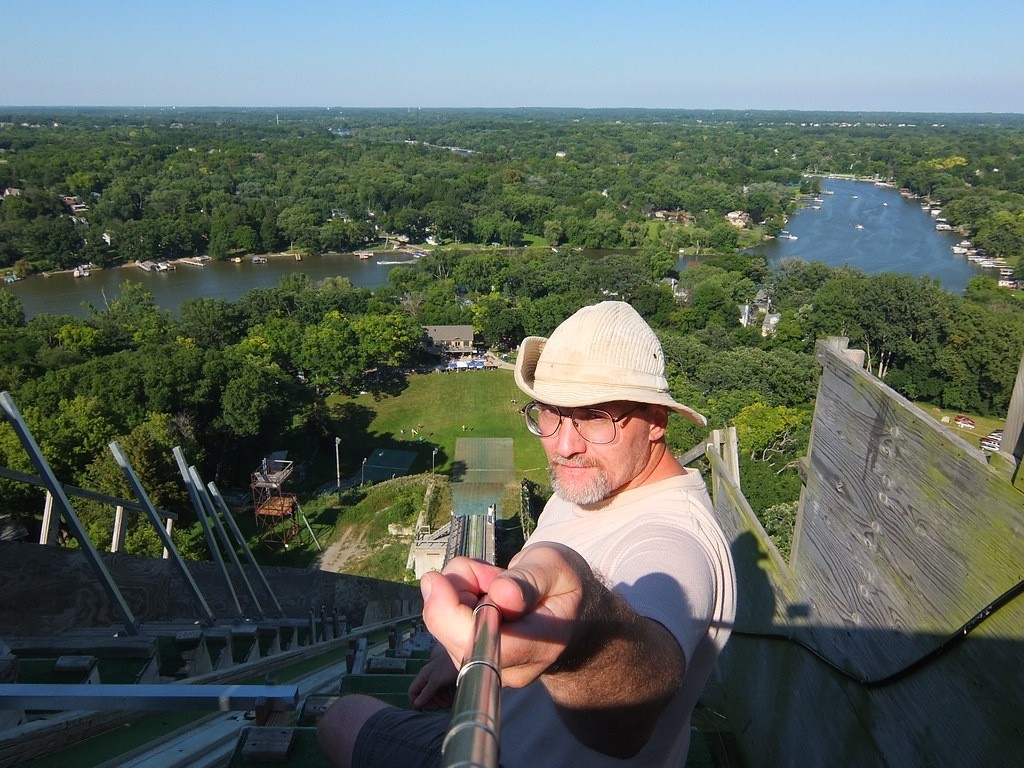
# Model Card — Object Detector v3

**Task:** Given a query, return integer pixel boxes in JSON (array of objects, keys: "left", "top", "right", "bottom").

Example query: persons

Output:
[
  {"left": 511, "top": 399, "right": 541, "bottom": 415},
  {"left": 399, "top": 422, "right": 466, "bottom": 447},
  {"left": 315, "top": 301, "right": 737, "bottom": 768}
]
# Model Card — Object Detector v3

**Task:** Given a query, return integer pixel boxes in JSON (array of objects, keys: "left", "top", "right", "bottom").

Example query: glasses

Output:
[{"left": 518, "top": 400, "right": 642, "bottom": 443}]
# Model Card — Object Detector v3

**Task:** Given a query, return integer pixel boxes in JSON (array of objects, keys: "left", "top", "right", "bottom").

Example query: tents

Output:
[{"left": 436, "top": 360, "right": 494, "bottom": 373}]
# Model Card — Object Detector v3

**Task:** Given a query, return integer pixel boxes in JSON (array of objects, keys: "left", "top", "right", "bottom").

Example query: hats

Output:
[{"left": 513, "top": 300, "right": 708, "bottom": 428}]
